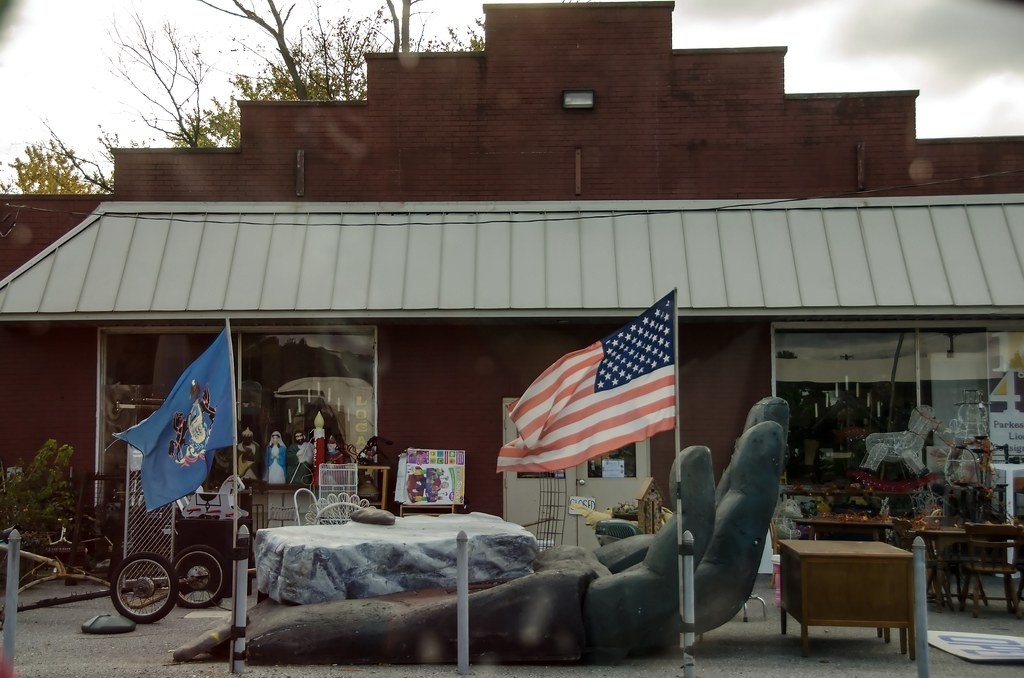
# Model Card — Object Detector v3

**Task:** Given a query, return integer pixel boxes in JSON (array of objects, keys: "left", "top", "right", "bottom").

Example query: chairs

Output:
[
  {"left": 769, "top": 519, "right": 777, "bottom": 590},
  {"left": 891, "top": 516, "right": 954, "bottom": 613},
  {"left": 957, "top": 525, "right": 1024, "bottom": 620},
  {"left": 264, "top": 504, "right": 302, "bottom": 527}
]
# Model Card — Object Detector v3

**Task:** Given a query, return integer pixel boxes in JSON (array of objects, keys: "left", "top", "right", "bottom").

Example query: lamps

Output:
[{"left": 562, "top": 88, "right": 596, "bottom": 109}]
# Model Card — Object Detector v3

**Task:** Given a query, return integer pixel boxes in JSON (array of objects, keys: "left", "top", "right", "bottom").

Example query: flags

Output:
[
  {"left": 495, "top": 288, "right": 678, "bottom": 472},
  {"left": 112, "top": 326, "right": 239, "bottom": 513}
]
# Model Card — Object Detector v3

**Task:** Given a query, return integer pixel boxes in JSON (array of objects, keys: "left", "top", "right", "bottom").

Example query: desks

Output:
[
  {"left": 791, "top": 514, "right": 893, "bottom": 542},
  {"left": 774, "top": 541, "right": 917, "bottom": 661},
  {"left": 914, "top": 525, "right": 989, "bottom": 615},
  {"left": 173, "top": 518, "right": 253, "bottom": 598}
]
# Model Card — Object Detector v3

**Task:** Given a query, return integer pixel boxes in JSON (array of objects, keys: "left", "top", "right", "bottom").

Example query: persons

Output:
[
  {"left": 237, "top": 426, "right": 262, "bottom": 479},
  {"left": 287, "top": 428, "right": 316, "bottom": 483},
  {"left": 326, "top": 434, "right": 345, "bottom": 465},
  {"left": 263, "top": 430, "right": 286, "bottom": 483}
]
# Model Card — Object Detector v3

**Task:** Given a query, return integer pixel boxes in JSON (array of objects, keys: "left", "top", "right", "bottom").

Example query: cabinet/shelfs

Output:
[{"left": 357, "top": 464, "right": 391, "bottom": 510}]
